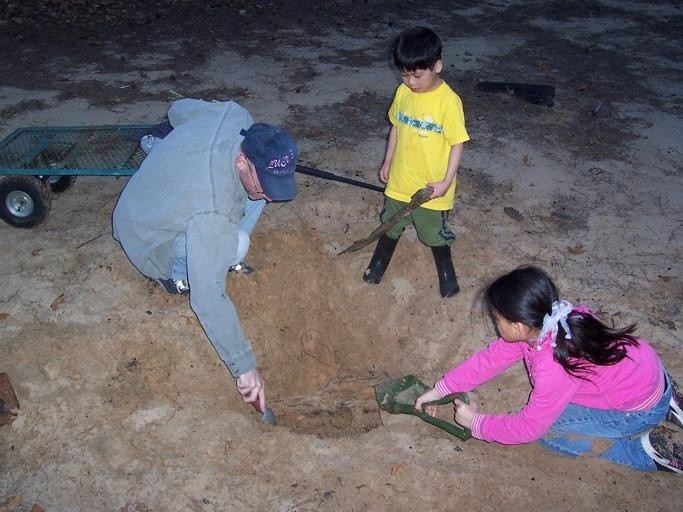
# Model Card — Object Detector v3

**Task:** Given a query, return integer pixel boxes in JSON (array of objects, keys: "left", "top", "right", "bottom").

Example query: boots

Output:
[
  {"left": 431, "top": 245, "right": 459, "bottom": 298},
  {"left": 363, "top": 232, "right": 399, "bottom": 284}
]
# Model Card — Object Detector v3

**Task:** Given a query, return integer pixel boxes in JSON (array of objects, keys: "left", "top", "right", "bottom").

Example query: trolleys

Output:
[{"left": 0, "top": 125, "right": 153, "bottom": 228}]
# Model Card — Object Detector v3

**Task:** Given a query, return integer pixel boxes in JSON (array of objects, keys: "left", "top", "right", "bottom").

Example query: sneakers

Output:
[
  {"left": 641, "top": 388, "right": 683, "bottom": 475},
  {"left": 229, "top": 260, "right": 252, "bottom": 274},
  {"left": 154, "top": 277, "right": 190, "bottom": 294}
]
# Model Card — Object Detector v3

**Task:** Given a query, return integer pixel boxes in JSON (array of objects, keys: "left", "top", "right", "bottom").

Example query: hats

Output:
[{"left": 240, "top": 123, "right": 297, "bottom": 201}]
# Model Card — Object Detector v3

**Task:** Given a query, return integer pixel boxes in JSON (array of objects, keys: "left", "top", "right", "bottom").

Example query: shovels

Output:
[
  {"left": 374, "top": 374, "right": 470, "bottom": 441},
  {"left": 337, "top": 186, "right": 434, "bottom": 256},
  {"left": 244, "top": 391, "right": 276, "bottom": 425}
]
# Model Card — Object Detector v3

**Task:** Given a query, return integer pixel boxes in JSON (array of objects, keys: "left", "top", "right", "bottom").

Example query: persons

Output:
[
  {"left": 413, "top": 264, "right": 682, "bottom": 475},
  {"left": 111, "top": 97, "right": 299, "bottom": 413},
  {"left": 360, "top": 24, "right": 471, "bottom": 298}
]
